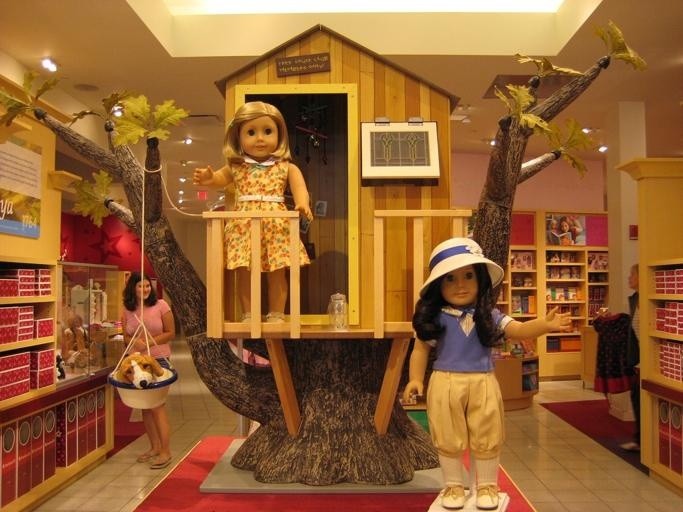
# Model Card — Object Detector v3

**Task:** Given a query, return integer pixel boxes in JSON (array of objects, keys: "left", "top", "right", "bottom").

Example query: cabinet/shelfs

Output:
[
  {"left": 0, "top": 255, "right": 58, "bottom": 409},
  {"left": 1, "top": 367, "right": 116, "bottom": 512},
  {"left": 494, "top": 265, "right": 510, "bottom": 318},
  {"left": 584, "top": 247, "right": 610, "bottom": 324},
  {"left": 541, "top": 244, "right": 588, "bottom": 356},
  {"left": 508, "top": 245, "right": 540, "bottom": 353},
  {"left": 637, "top": 255, "right": 682, "bottom": 498},
  {"left": 490, "top": 350, "right": 540, "bottom": 413}
]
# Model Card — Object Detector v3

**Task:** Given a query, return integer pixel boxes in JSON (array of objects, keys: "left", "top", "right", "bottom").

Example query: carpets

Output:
[
  {"left": 132, "top": 434, "right": 537, "bottom": 511},
  {"left": 538, "top": 397, "right": 650, "bottom": 476}
]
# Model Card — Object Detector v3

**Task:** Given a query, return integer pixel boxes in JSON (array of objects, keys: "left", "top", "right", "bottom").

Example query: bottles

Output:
[{"left": 327, "top": 292, "right": 349, "bottom": 331}]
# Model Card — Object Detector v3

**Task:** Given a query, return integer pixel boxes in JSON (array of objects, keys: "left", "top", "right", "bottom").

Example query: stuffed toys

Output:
[{"left": 116, "top": 353, "right": 164, "bottom": 388}]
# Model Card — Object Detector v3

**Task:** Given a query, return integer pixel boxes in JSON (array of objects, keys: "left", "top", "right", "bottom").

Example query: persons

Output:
[
  {"left": 193, "top": 101, "right": 313, "bottom": 324},
  {"left": 123, "top": 272, "right": 177, "bottom": 468},
  {"left": 549, "top": 215, "right": 582, "bottom": 245},
  {"left": 401, "top": 238, "right": 573, "bottom": 509},
  {"left": 618, "top": 263, "right": 640, "bottom": 454}
]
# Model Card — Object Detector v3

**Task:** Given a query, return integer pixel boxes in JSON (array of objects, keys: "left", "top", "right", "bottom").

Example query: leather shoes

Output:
[
  {"left": 476, "top": 484, "right": 499, "bottom": 510},
  {"left": 441, "top": 487, "right": 466, "bottom": 509}
]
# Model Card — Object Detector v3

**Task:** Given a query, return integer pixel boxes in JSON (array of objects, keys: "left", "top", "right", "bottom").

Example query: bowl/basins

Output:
[{"left": 105, "top": 366, "right": 178, "bottom": 408}]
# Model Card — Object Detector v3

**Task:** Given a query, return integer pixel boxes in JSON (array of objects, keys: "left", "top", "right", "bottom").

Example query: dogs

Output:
[{"left": 114, "top": 351, "right": 173, "bottom": 391}]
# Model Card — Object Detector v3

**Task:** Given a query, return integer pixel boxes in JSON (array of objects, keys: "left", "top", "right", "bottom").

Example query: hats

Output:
[{"left": 418, "top": 236, "right": 505, "bottom": 299}]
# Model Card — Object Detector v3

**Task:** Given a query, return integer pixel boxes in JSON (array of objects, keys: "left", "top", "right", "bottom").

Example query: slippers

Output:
[
  {"left": 137, "top": 451, "right": 159, "bottom": 463},
  {"left": 150, "top": 455, "right": 174, "bottom": 469}
]
